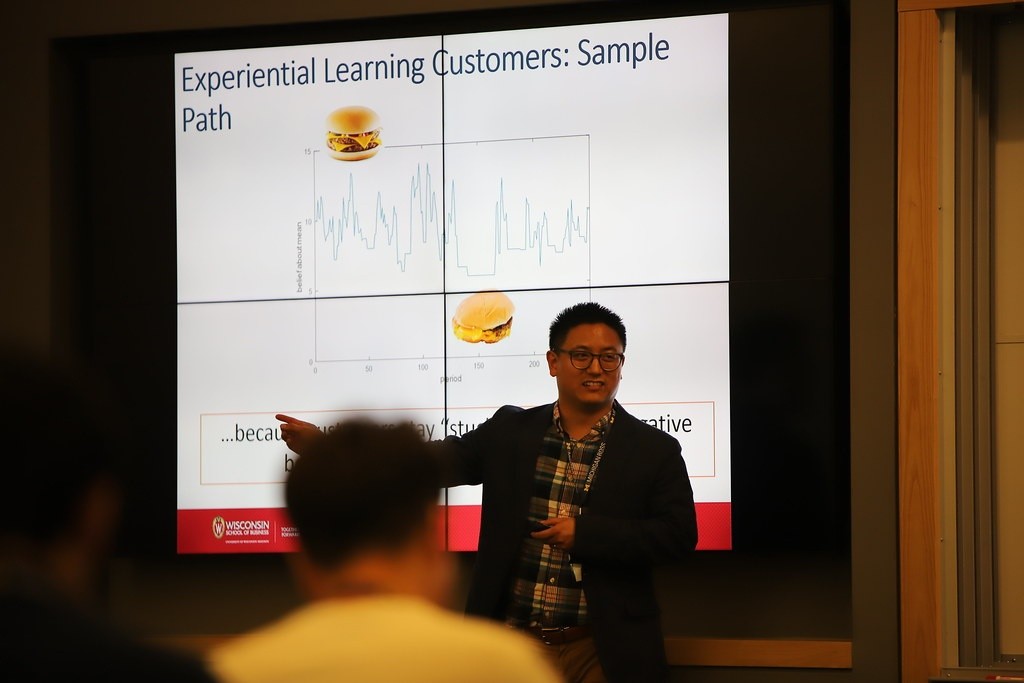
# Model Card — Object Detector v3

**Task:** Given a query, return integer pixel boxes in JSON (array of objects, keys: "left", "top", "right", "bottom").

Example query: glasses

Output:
[{"left": 552, "top": 347, "right": 625, "bottom": 372}]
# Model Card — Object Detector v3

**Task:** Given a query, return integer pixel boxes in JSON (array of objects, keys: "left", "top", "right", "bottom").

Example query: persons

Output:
[
  {"left": 207, "top": 419, "right": 562, "bottom": 683},
  {"left": 275, "top": 303, "right": 698, "bottom": 683},
  {"left": 0, "top": 355, "right": 221, "bottom": 683}
]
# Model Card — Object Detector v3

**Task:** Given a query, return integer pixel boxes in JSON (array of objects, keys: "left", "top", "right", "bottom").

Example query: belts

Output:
[{"left": 528, "top": 624, "right": 595, "bottom": 645}]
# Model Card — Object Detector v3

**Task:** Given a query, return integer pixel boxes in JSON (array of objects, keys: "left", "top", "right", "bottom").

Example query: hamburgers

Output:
[
  {"left": 326, "top": 106, "right": 383, "bottom": 161},
  {"left": 452, "top": 290, "right": 514, "bottom": 344}
]
